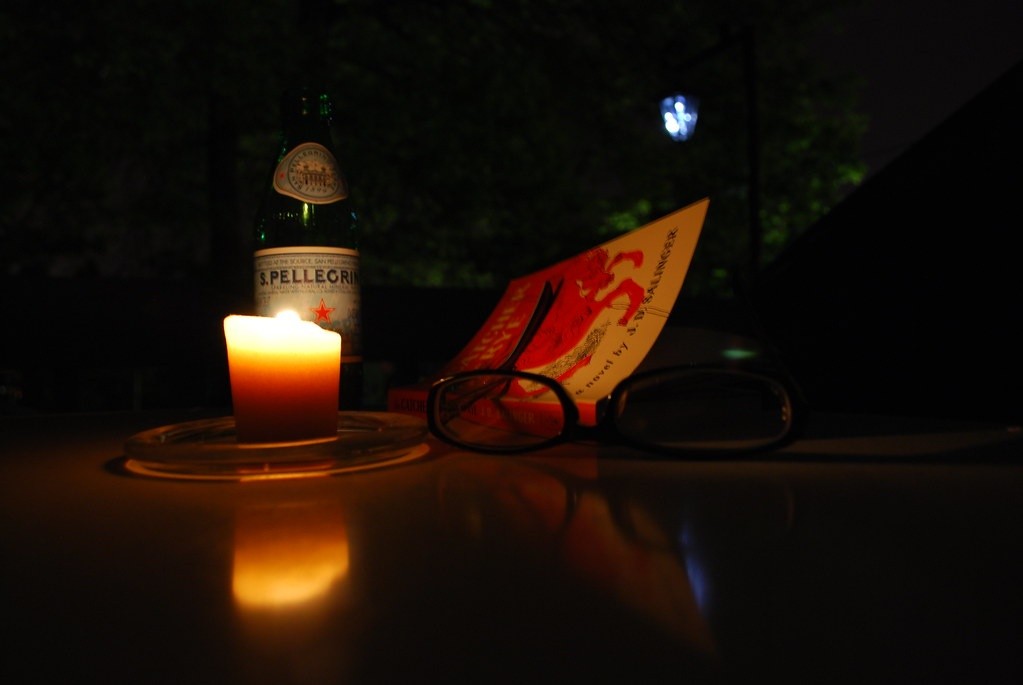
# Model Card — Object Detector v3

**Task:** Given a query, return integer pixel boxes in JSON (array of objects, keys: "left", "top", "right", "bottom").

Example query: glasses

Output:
[{"left": 426, "top": 281, "right": 800, "bottom": 455}]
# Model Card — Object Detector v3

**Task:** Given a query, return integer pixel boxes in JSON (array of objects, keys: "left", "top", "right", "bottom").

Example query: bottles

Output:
[{"left": 251, "top": 82, "right": 371, "bottom": 414}]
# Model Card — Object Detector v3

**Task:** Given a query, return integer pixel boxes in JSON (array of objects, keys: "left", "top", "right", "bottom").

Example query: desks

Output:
[{"left": 0, "top": 404, "right": 1022, "bottom": 685}]
